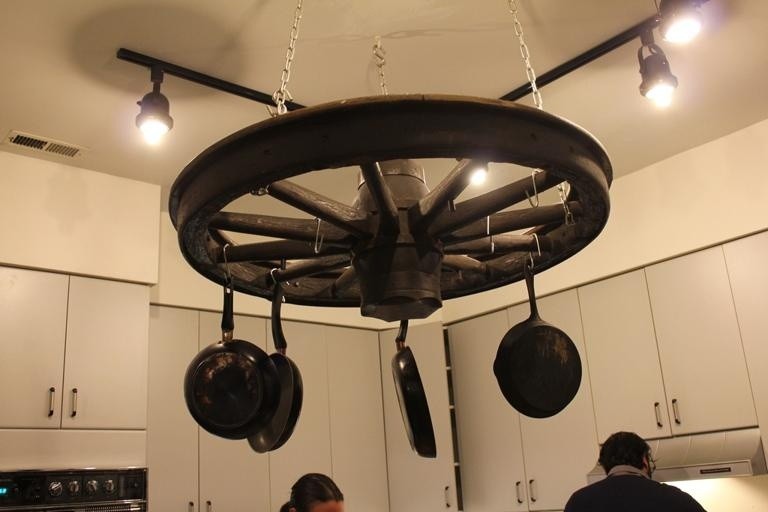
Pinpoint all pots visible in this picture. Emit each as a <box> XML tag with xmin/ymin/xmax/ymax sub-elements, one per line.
<box><xmin>491</xmin><ymin>255</ymin><xmax>585</xmax><ymax>420</ymax></box>
<box><xmin>389</xmin><ymin>317</ymin><xmax>442</xmax><ymax>459</ymax></box>
<box><xmin>181</xmin><ymin>276</ymin><xmax>283</xmax><ymax>440</ymax></box>
<box><xmin>246</xmin><ymin>280</ymin><xmax>305</xmax><ymax>455</ymax></box>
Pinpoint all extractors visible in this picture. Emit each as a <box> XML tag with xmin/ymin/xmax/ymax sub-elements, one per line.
<box><xmin>584</xmin><ymin>425</ymin><xmax>767</xmax><ymax>485</ymax></box>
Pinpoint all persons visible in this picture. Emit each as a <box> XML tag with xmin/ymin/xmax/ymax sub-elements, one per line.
<box><xmin>564</xmin><ymin>431</ymin><xmax>708</xmax><ymax>511</ymax></box>
<box><xmin>279</xmin><ymin>470</ymin><xmax>346</xmax><ymax>512</ymax></box>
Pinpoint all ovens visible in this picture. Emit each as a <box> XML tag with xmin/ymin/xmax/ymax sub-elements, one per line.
<box><xmin>0</xmin><ymin>468</ymin><xmax>148</xmax><ymax>511</ymax></box>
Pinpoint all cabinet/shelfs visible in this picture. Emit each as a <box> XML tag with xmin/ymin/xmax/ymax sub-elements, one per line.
<box><xmin>147</xmin><ymin>303</ymin><xmax>271</xmax><ymax>512</ymax></box>
<box><xmin>266</xmin><ymin>318</ymin><xmax>391</xmax><ymax>512</ymax></box>
<box><xmin>577</xmin><ymin>244</ymin><xmax>758</xmax><ymax>445</ymax></box>
<box><xmin>380</xmin><ymin>321</ymin><xmax>459</xmax><ymax>511</ymax></box>
<box><xmin>0</xmin><ymin>264</ymin><xmax>151</xmax><ymax>471</ymax></box>
<box><xmin>722</xmin><ymin>230</ymin><xmax>768</xmax><ymax>472</ymax></box>
<box><xmin>448</xmin><ymin>289</ymin><xmax>606</xmax><ymax>512</ymax></box>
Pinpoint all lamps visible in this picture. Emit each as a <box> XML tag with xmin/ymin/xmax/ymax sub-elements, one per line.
<box><xmin>116</xmin><ymin>0</ymin><xmax>708</xmax><ymax>185</ymax></box>
